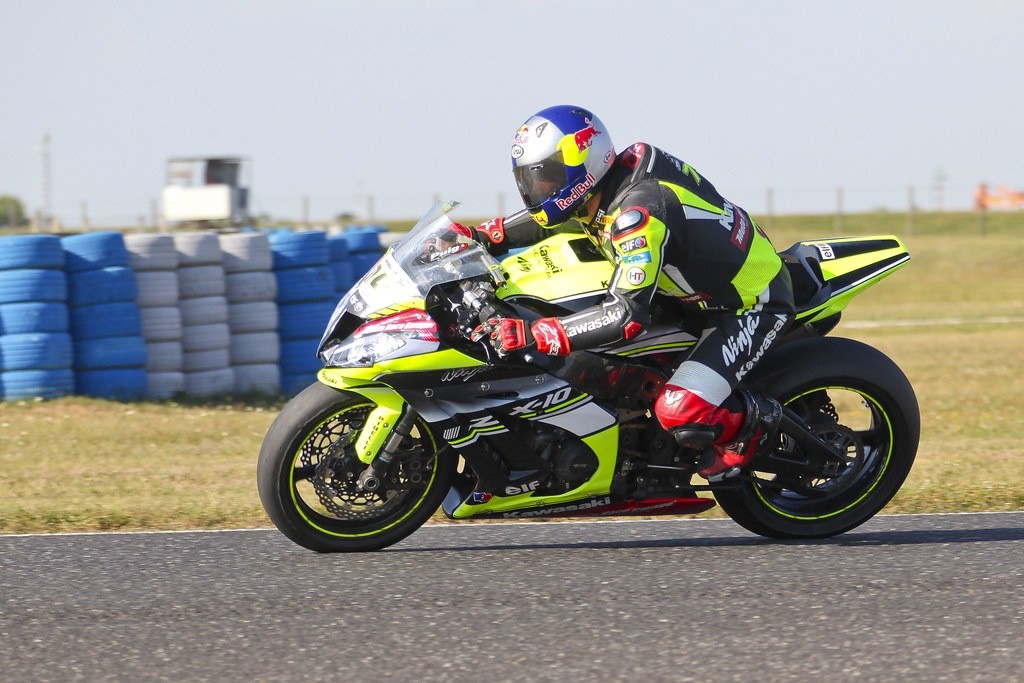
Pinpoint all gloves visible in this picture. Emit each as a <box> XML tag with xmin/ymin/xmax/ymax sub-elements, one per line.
<box><xmin>471</xmin><ymin>317</ymin><xmax>570</xmax><ymax>360</ymax></box>
<box><xmin>422</xmin><ymin>217</ymin><xmax>509</xmax><ymax>264</ymax></box>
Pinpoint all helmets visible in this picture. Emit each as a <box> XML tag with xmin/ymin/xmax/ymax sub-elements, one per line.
<box><xmin>511</xmin><ymin>105</ymin><xmax>615</xmax><ymax>229</ymax></box>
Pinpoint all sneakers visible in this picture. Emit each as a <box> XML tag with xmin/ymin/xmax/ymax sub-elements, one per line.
<box><xmin>697</xmin><ymin>389</ymin><xmax>782</xmax><ymax>482</ymax></box>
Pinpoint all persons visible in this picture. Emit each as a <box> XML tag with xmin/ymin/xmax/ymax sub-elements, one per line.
<box><xmin>425</xmin><ymin>104</ymin><xmax>798</xmax><ymax>483</ymax></box>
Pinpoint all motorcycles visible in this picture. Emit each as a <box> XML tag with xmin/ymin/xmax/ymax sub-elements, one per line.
<box><xmin>254</xmin><ymin>189</ymin><xmax>925</xmax><ymax>557</ymax></box>
